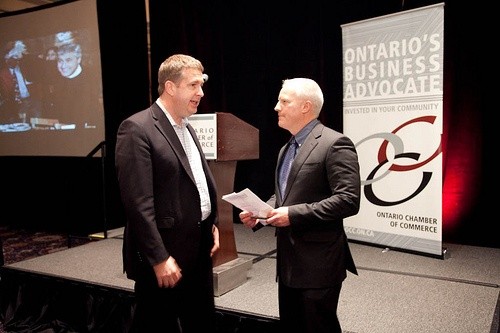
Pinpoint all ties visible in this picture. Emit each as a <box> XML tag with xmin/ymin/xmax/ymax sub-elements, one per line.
<box><xmin>279</xmin><ymin>137</ymin><xmax>297</xmax><ymax>202</ymax></box>
<box><xmin>12</xmin><ymin>72</ymin><xmax>23</xmax><ymax>103</ymax></box>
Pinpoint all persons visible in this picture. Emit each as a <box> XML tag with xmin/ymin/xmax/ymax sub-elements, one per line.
<box><xmin>43</xmin><ymin>47</ymin><xmax>59</xmax><ymax>60</ymax></box>
<box><xmin>116</xmin><ymin>53</ymin><xmax>222</xmax><ymax>333</ymax></box>
<box><xmin>38</xmin><ymin>44</ymin><xmax>99</xmax><ymax>128</ymax></box>
<box><xmin>0</xmin><ymin>41</ymin><xmax>37</xmax><ymax>126</ymax></box>
<box><xmin>238</xmin><ymin>78</ymin><xmax>361</xmax><ymax>333</ymax></box>
